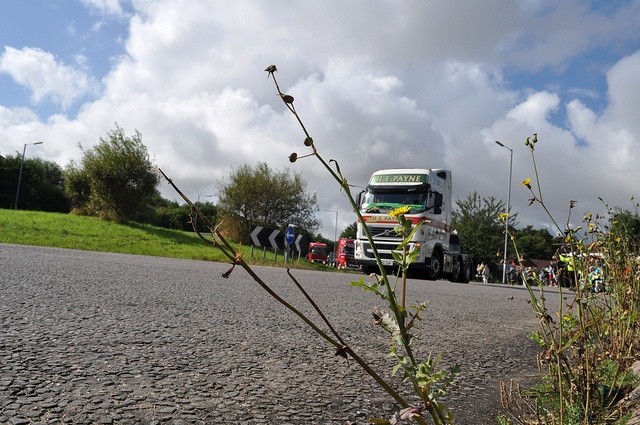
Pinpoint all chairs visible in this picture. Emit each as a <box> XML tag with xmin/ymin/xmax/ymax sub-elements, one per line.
<box><xmin>579</xmin><ymin>271</ymin><xmax>605</xmax><ymax>292</ymax></box>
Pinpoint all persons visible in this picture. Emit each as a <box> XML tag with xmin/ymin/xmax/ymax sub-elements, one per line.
<box><xmin>474</xmin><ymin>252</ymin><xmax>606</xmax><ymax>295</ymax></box>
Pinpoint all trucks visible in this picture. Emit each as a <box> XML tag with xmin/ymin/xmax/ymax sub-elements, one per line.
<box><xmin>305</xmin><ymin>242</ymin><xmax>330</xmax><ymax>267</ymax></box>
<box><xmin>354</xmin><ymin>168</ymin><xmax>476</xmax><ymax>284</ymax></box>
<box><xmin>329</xmin><ymin>238</ymin><xmax>360</xmax><ymax>270</ymax></box>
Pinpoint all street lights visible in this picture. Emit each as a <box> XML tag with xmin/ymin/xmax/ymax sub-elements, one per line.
<box><xmin>194</xmin><ymin>194</ymin><xmax>212</xmax><ymax>232</ymax></box>
<box><xmin>495</xmin><ymin>140</ymin><xmax>513</xmax><ymax>284</ymax></box>
<box><xmin>14</xmin><ymin>141</ymin><xmax>44</xmax><ymax>210</ymax></box>
<box><xmin>324</xmin><ymin>209</ymin><xmax>338</xmax><ymax>262</ymax></box>
